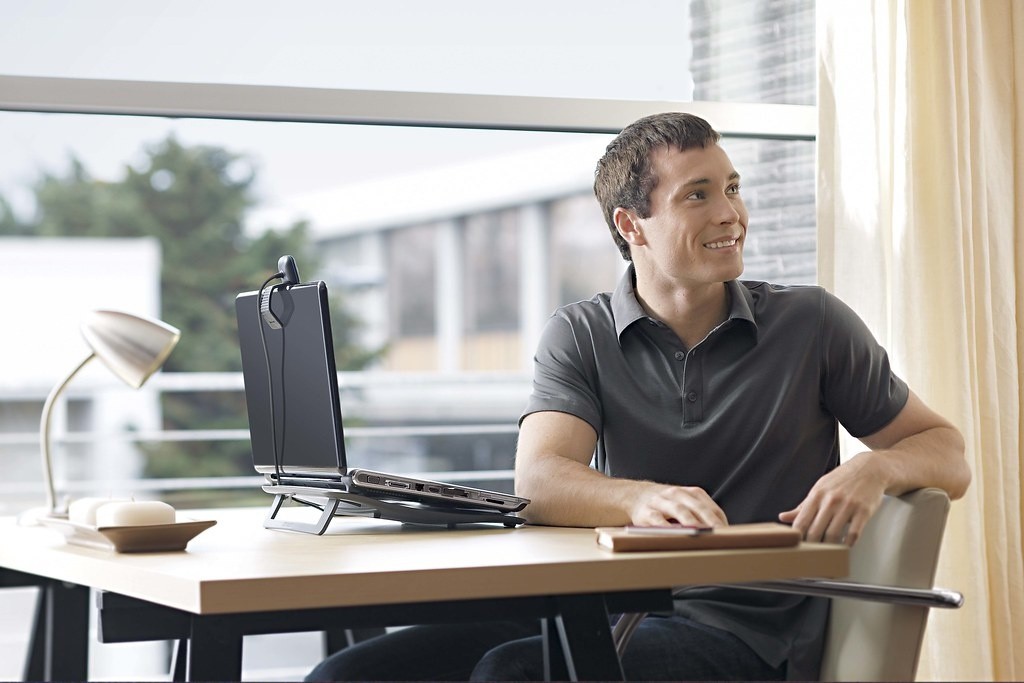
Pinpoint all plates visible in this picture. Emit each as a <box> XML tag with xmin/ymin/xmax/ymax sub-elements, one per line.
<box><xmin>42</xmin><ymin>513</ymin><xmax>218</xmax><ymax>553</ymax></box>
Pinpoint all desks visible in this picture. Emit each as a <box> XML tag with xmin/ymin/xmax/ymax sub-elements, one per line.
<box><xmin>0</xmin><ymin>510</ymin><xmax>848</xmax><ymax>683</ymax></box>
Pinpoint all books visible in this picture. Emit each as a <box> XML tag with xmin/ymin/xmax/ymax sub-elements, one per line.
<box><xmin>594</xmin><ymin>522</ymin><xmax>801</xmax><ymax>550</ymax></box>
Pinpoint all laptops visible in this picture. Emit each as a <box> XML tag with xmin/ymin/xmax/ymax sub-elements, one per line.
<box><xmin>236</xmin><ymin>281</ymin><xmax>532</xmax><ymax>516</ymax></box>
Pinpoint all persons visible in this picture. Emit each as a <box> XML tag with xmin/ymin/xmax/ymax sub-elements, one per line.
<box><xmin>304</xmin><ymin>112</ymin><xmax>973</xmax><ymax>683</ymax></box>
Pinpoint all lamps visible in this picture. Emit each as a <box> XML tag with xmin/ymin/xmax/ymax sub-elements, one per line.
<box><xmin>17</xmin><ymin>310</ymin><xmax>181</xmax><ymax>528</ymax></box>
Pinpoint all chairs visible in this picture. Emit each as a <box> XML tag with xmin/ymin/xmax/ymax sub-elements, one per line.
<box><xmin>822</xmin><ymin>489</ymin><xmax>952</xmax><ymax>683</ymax></box>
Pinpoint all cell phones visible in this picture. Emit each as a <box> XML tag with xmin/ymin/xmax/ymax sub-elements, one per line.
<box><xmin>626</xmin><ymin>524</ymin><xmax>713</xmax><ymax>535</ymax></box>
